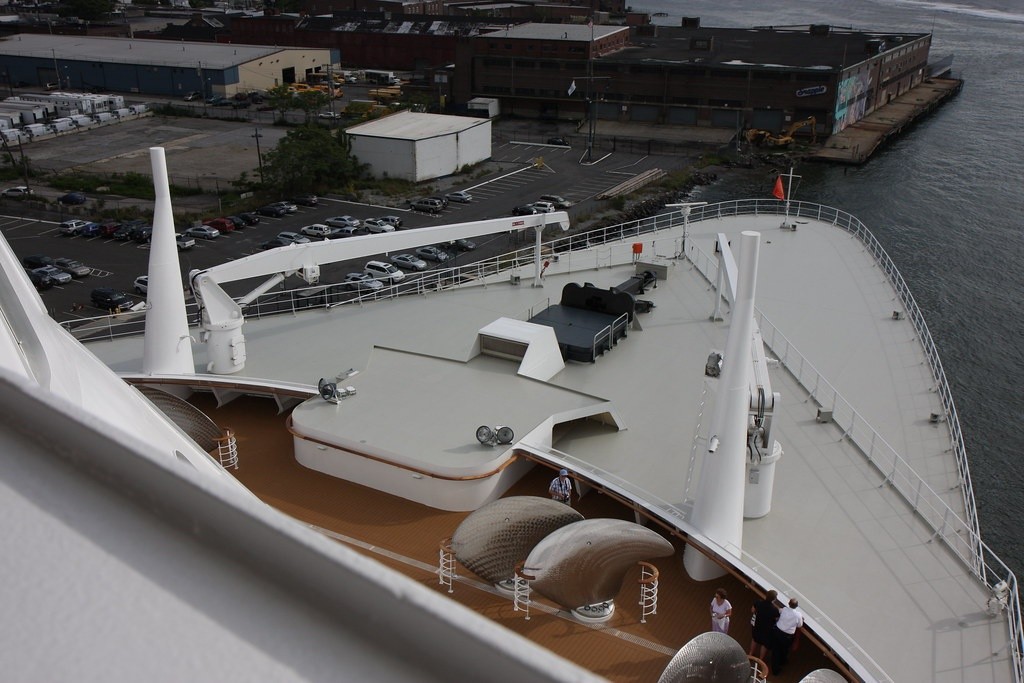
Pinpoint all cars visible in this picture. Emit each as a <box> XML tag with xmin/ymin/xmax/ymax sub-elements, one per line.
<box><xmin>293</xmin><ymin>193</ymin><xmax>317</xmax><ymax>205</ymax></box>
<box><xmin>23</xmin><ymin>256</ymin><xmax>46</xmax><ymax>269</ymax></box>
<box><xmin>89</xmin><ymin>275</ymin><xmax>149</xmax><ymax>311</ymax></box>
<box><xmin>238</xmin><ymin>213</ymin><xmax>259</xmax><ymax>225</ymax></box>
<box><xmin>256</xmin><ymin>205</ymin><xmax>286</xmax><ymax>218</ymax></box>
<box><xmin>184</xmin><ymin>70</ymin><xmax>570</xmax><ymax>146</ymax></box>
<box><xmin>186</xmin><ymin>225</ymin><xmax>220</xmax><ymax>239</ymax></box>
<box><xmin>202</xmin><ymin>218</ymin><xmax>235</xmax><ymax>234</ymax></box>
<box><xmin>99</xmin><ymin>222</ymin><xmax>121</xmax><ymax>237</ymax></box>
<box><xmin>271</xmin><ymin>201</ymin><xmax>298</xmax><ymax>213</ymax></box>
<box><xmin>261</xmin><ymin>192</ymin><xmax>571</xmax><ymax>292</ymax></box>
<box><xmin>34</xmin><ymin>266</ymin><xmax>72</xmax><ymax>285</ymax></box>
<box><xmin>1</xmin><ymin>186</ymin><xmax>100</xmax><ymax>237</ymax></box>
<box><xmin>226</xmin><ymin>216</ymin><xmax>247</xmax><ymax>229</ymax></box>
<box><xmin>54</xmin><ymin>258</ymin><xmax>90</xmax><ymax>279</ymax></box>
<box><xmin>113</xmin><ymin>224</ymin><xmax>152</xmax><ymax>243</ymax></box>
<box><xmin>29</xmin><ymin>273</ymin><xmax>53</xmax><ymax>290</ymax></box>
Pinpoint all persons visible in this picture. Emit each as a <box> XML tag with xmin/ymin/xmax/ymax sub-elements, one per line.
<box><xmin>709</xmin><ymin>588</ymin><xmax>732</xmax><ymax>633</ymax></box>
<box><xmin>771</xmin><ymin>598</ymin><xmax>804</xmax><ymax>676</ymax></box>
<box><xmin>748</xmin><ymin>590</ymin><xmax>780</xmax><ymax>668</ymax></box>
<box><xmin>549</xmin><ymin>469</ymin><xmax>572</xmax><ymax>508</ymax></box>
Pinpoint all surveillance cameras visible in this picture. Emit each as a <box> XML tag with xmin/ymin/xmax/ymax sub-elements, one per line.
<box><xmin>709</xmin><ymin>438</ymin><xmax>718</xmax><ymax>452</ymax></box>
<box><xmin>130</xmin><ymin>302</ymin><xmax>146</xmax><ymax>313</ymax></box>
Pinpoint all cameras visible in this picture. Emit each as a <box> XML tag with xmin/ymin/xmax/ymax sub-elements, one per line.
<box><xmin>712</xmin><ymin>613</ymin><xmax>717</xmax><ymax>616</ymax></box>
<box><xmin>562</xmin><ymin>494</ymin><xmax>569</xmax><ymax>501</ymax></box>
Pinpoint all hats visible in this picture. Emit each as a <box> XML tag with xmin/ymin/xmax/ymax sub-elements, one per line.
<box><xmin>560</xmin><ymin>470</ymin><xmax>568</xmax><ymax>475</ymax></box>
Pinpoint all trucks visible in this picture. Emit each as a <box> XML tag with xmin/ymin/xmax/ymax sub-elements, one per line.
<box><xmin>0</xmin><ymin>80</ymin><xmax>151</xmax><ymax>144</ymax></box>
<box><xmin>175</xmin><ymin>233</ymin><xmax>196</xmax><ymax>251</ymax></box>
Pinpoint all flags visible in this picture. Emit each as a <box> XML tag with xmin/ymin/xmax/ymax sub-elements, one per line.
<box><xmin>568</xmin><ymin>81</ymin><xmax>576</xmax><ymax>96</ymax></box>
<box><xmin>772</xmin><ymin>175</ymin><xmax>784</xmax><ymax>201</ymax></box>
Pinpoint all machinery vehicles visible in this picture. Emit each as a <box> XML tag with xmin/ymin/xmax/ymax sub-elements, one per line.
<box><xmin>745</xmin><ymin>116</ymin><xmax>817</xmax><ymax>150</ymax></box>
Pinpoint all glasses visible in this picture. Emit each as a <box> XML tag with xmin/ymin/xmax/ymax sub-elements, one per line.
<box><xmin>561</xmin><ymin>475</ymin><xmax>567</xmax><ymax>477</ymax></box>
<box><xmin>715</xmin><ymin>597</ymin><xmax>718</xmax><ymax>599</ymax></box>
